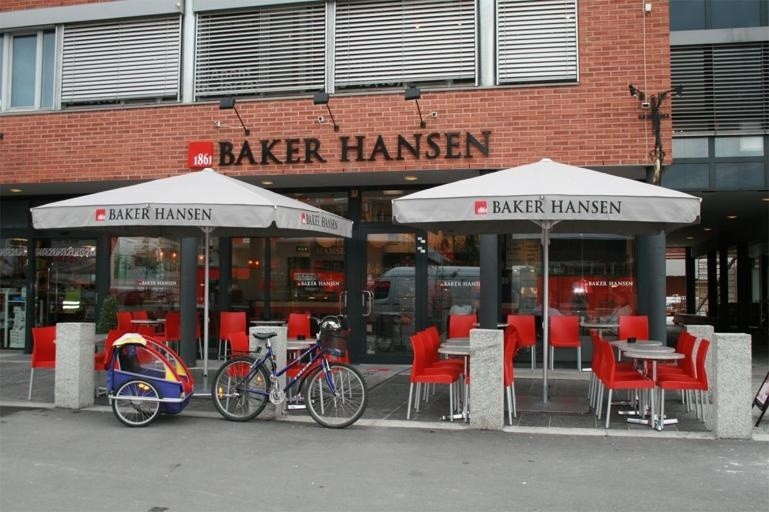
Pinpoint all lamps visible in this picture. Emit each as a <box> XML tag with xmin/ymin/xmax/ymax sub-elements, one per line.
<box><xmin>405</xmin><ymin>85</ymin><xmax>426</xmax><ymax>129</ymax></box>
<box><xmin>314</xmin><ymin>93</ymin><xmax>339</xmax><ymax>131</ymax></box>
<box><xmin>220</xmin><ymin>97</ymin><xmax>250</xmax><ymax>135</ymax></box>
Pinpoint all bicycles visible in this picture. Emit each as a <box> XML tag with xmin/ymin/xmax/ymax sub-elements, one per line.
<box><xmin>213</xmin><ymin>317</ymin><xmax>370</xmax><ymax>429</ymax></box>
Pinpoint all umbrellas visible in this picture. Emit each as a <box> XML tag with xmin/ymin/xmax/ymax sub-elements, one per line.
<box><xmin>392</xmin><ymin>159</ymin><xmax>702</xmax><ymax>414</ymax></box>
<box><xmin>30</xmin><ymin>168</ymin><xmax>353</xmax><ymax>397</ymax></box>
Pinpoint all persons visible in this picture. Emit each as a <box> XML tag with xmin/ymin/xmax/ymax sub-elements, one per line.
<box><xmin>449</xmin><ymin>295</ymin><xmax>473</xmax><ymax>316</ymax></box>
<box><xmin>530</xmin><ymin>293</ymin><xmax>565</xmax><ymax>317</ymax></box>
<box><xmin>606</xmin><ymin>291</ymin><xmax>634</xmax><ymax>336</ymax></box>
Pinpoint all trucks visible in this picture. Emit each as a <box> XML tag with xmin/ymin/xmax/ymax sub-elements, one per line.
<box><xmin>48</xmin><ymin>236</ymin><xmax>182</xmax><ymax>305</ymax></box>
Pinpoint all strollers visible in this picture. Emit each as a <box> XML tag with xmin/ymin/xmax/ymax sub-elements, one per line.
<box><xmin>101</xmin><ymin>332</ymin><xmax>198</xmax><ymax>422</ymax></box>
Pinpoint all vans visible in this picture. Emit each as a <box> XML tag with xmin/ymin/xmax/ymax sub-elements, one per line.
<box><xmin>367</xmin><ymin>265</ymin><xmax>539</xmax><ymax>350</ymax></box>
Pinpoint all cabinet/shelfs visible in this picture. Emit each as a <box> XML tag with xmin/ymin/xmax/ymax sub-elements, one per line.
<box><xmin>0</xmin><ymin>287</ymin><xmax>26</xmax><ymax>349</ymax></box>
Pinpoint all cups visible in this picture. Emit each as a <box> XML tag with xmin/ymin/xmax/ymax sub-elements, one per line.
<box><xmin>297</xmin><ymin>335</ymin><xmax>305</xmax><ymax>340</ymax></box>
<box><xmin>151</xmin><ymin>317</ymin><xmax>157</xmax><ymax>320</ymax></box>
<box><xmin>626</xmin><ymin>336</ymin><xmax>635</xmax><ymax>343</ymax></box>
